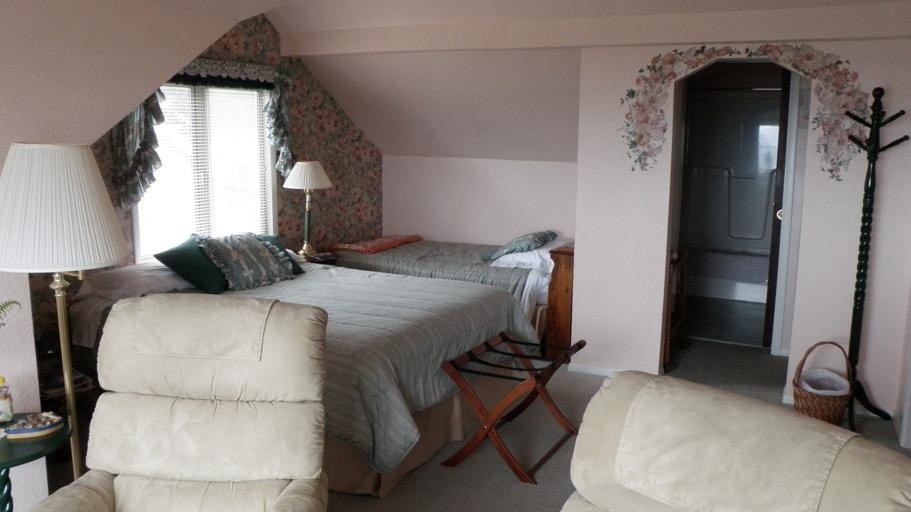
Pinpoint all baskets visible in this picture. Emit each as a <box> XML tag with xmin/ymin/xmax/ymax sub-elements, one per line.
<box><xmin>791</xmin><ymin>341</ymin><xmax>854</xmax><ymax>425</ymax></box>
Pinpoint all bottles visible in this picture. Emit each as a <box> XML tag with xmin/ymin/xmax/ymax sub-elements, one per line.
<box><xmin>0</xmin><ymin>377</ymin><xmax>15</xmax><ymax>424</ymax></box>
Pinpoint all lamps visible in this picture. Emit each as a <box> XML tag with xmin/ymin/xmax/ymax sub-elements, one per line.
<box><xmin>1</xmin><ymin>140</ymin><xmax>131</xmax><ymax>480</ymax></box>
<box><xmin>283</xmin><ymin>160</ymin><xmax>334</xmax><ymax>255</ymax></box>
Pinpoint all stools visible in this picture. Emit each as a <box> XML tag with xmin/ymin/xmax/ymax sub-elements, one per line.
<box><xmin>437</xmin><ymin>328</ymin><xmax>590</xmax><ymax>487</ymax></box>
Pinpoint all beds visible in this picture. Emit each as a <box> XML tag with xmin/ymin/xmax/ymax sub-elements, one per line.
<box><xmin>70</xmin><ymin>232</ymin><xmax>540</xmax><ymax>494</ymax></box>
<box><xmin>311</xmin><ymin>233</ymin><xmax>573</xmax><ymax>350</ymax></box>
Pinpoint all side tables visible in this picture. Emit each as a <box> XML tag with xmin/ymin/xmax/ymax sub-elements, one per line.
<box><xmin>2</xmin><ymin>412</ymin><xmax>73</xmax><ymax>512</ymax></box>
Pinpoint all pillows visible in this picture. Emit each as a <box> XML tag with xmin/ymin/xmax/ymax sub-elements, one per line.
<box><xmin>488</xmin><ymin>229</ymin><xmax>557</xmax><ymax>263</ymax></box>
<box><xmin>152</xmin><ymin>232</ymin><xmax>306</xmax><ymax>293</ymax></box>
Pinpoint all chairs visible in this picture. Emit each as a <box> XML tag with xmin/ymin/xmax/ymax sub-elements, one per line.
<box><xmin>23</xmin><ymin>290</ymin><xmax>336</xmax><ymax>512</ymax></box>
